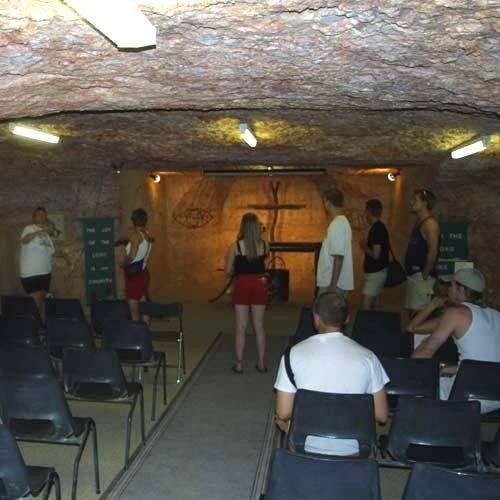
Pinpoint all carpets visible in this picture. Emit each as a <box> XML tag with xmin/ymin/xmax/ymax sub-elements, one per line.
<box><xmin>93</xmin><ymin>328</ymin><xmax>298</xmax><ymax>500</ymax></box>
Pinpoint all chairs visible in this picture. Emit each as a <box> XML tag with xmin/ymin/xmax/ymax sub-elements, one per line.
<box><xmin>263</xmin><ymin>309</ymin><xmax>500</xmax><ymax>499</ymax></box>
<box><xmin>0</xmin><ymin>294</ymin><xmax>188</xmax><ymax>499</ymax></box>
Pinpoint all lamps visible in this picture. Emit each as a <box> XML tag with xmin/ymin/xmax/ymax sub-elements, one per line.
<box><xmin>448</xmin><ymin>132</ymin><xmax>491</xmax><ymax>160</ymax></box>
<box><xmin>55</xmin><ymin>0</ymin><xmax>161</xmax><ymax>53</ymax></box>
<box><xmin>8</xmin><ymin>121</ymin><xmax>60</xmax><ymax>149</ymax></box>
<box><xmin>238</xmin><ymin>119</ymin><xmax>258</xmax><ymax>149</ymax></box>
<box><xmin>386</xmin><ymin>169</ymin><xmax>401</xmax><ymax>183</ymax></box>
<box><xmin>147</xmin><ymin>170</ymin><xmax>162</xmax><ymax>185</ymax></box>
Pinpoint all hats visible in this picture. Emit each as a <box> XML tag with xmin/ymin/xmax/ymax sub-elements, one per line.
<box><xmin>441</xmin><ymin>268</ymin><xmax>486</xmax><ymax>293</ymax></box>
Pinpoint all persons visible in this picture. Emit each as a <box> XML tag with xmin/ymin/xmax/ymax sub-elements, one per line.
<box><xmin>403</xmin><ymin>188</ymin><xmax>441</xmax><ymax>323</ymax></box>
<box><xmin>406</xmin><ymin>268</ymin><xmax>500</xmax><ymax>413</ymax></box>
<box><xmin>18</xmin><ymin>207</ymin><xmax>56</xmax><ymax>323</ymax></box>
<box><xmin>272</xmin><ymin>292</ymin><xmax>389</xmax><ymax>438</ymax></box>
<box><xmin>226</xmin><ymin>213</ymin><xmax>272</xmax><ymax>373</ymax></box>
<box><xmin>119</xmin><ymin>207</ymin><xmax>154</xmax><ymax>329</ymax></box>
<box><xmin>316</xmin><ymin>189</ymin><xmax>355</xmax><ymax>306</ymax></box>
<box><xmin>358</xmin><ymin>198</ymin><xmax>390</xmax><ymax>312</ymax></box>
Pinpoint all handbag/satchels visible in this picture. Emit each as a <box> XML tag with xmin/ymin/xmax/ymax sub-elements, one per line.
<box><xmin>124</xmin><ymin>258</ymin><xmax>143</xmax><ymax>276</ymax></box>
<box><xmin>384</xmin><ymin>261</ymin><xmax>407</xmax><ymax>287</ymax></box>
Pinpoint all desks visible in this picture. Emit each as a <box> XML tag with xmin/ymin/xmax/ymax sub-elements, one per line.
<box><xmin>266</xmin><ymin>241</ymin><xmax>324</xmax><ymax>306</ymax></box>
